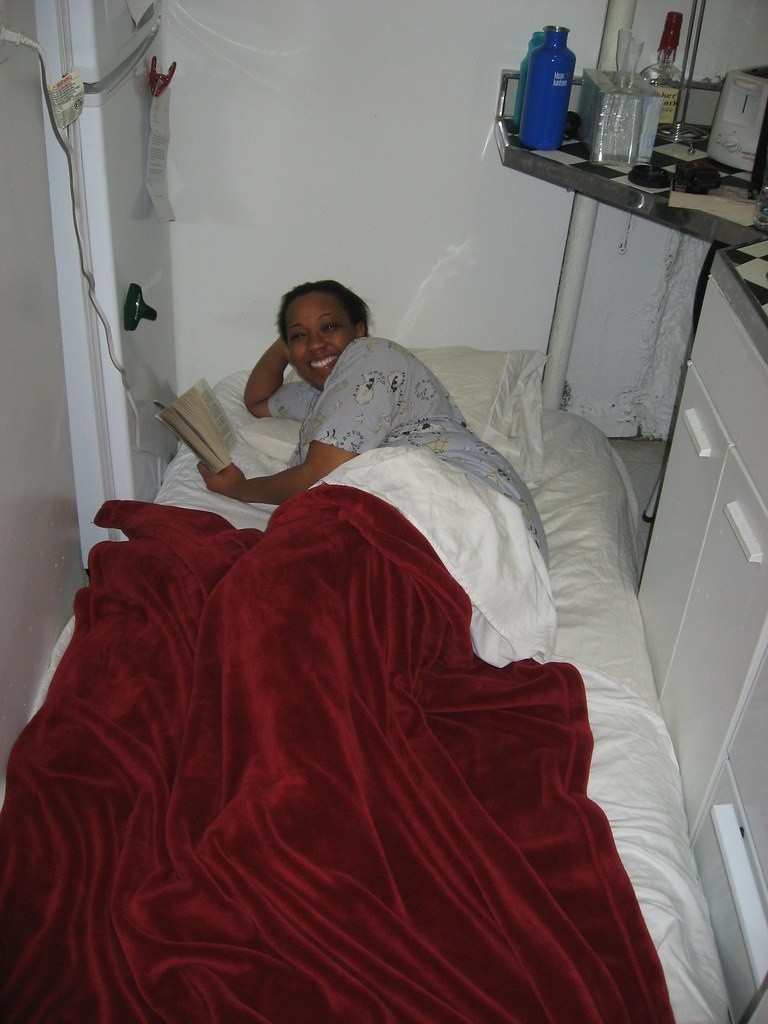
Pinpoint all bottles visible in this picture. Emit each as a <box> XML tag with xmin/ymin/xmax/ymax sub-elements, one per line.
<box><xmin>639</xmin><ymin>12</ymin><xmax>684</xmax><ymax>136</ymax></box>
<box><xmin>518</xmin><ymin>25</ymin><xmax>576</xmax><ymax>150</ymax></box>
<box><xmin>513</xmin><ymin>32</ymin><xmax>546</xmax><ymax>133</ymax></box>
<box><xmin>753</xmin><ymin>162</ymin><xmax>768</xmax><ymax>233</ymax></box>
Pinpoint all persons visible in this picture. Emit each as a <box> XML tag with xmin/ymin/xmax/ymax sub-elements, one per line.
<box><xmin>196</xmin><ymin>280</ymin><xmax>558</xmax><ymax>667</ymax></box>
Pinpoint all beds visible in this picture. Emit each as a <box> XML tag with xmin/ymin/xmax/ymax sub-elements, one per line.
<box><xmin>0</xmin><ymin>409</ymin><xmax>728</xmax><ymax>1024</ymax></box>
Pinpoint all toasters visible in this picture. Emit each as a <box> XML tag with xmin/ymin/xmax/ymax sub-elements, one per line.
<box><xmin>706</xmin><ymin>64</ymin><xmax>768</xmax><ymax>171</ymax></box>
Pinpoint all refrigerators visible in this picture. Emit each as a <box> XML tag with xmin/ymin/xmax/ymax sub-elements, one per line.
<box><xmin>35</xmin><ymin>0</ymin><xmax>183</xmax><ymax>576</ymax></box>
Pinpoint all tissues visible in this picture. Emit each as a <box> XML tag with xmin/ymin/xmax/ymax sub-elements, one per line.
<box><xmin>576</xmin><ymin>30</ymin><xmax>665</xmax><ymax>166</ymax></box>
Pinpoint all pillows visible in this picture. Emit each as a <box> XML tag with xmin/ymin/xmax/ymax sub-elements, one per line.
<box><xmin>235</xmin><ymin>346</ymin><xmax>547</xmax><ymax>490</ymax></box>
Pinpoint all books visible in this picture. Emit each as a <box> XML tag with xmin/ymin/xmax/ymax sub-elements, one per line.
<box><xmin>155</xmin><ymin>377</ymin><xmax>238</xmax><ymax>475</ymax></box>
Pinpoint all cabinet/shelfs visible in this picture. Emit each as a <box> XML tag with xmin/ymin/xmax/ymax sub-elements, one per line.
<box><xmin>626</xmin><ymin>275</ymin><xmax>768</xmax><ymax>1023</ymax></box>
